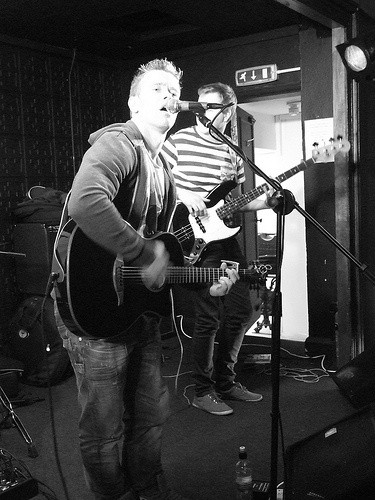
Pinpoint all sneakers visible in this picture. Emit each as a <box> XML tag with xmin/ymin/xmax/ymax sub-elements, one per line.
<box><xmin>192</xmin><ymin>392</ymin><xmax>233</xmax><ymax>415</ymax></box>
<box><xmin>221</xmin><ymin>382</ymin><xmax>263</xmax><ymax>401</ymax></box>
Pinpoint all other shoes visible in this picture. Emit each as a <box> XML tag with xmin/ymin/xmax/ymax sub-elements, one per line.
<box><xmin>118</xmin><ymin>472</ymin><xmax>189</xmax><ymax>500</ymax></box>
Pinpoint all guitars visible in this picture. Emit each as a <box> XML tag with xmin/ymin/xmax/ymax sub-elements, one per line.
<box><xmin>53</xmin><ymin>218</ymin><xmax>267</xmax><ymax>340</ymax></box>
<box><xmin>168</xmin><ymin>134</ymin><xmax>351</xmax><ymax>267</ymax></box>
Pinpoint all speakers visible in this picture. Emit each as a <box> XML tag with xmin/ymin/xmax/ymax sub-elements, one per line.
<box><xmin>280</xmin><ymin>344</ymin><xmax>375</xmax><ymax>500</ymax></box>
<box><xmin>14</xmin><ymin>223</ymin><xmax>63</xmax><ymax>295</ymax></box>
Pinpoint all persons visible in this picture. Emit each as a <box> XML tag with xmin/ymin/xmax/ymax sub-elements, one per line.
<box><xmin>48</xmin><ymin>59</ymin><xmax>242</xmax><ymax>499</ymax></box>
<box><xmin>160</xmin><ymin>82</ymin><xmax>294</xmax><ymax>416</ymax></box>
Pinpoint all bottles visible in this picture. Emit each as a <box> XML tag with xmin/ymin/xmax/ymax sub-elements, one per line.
<box><xmin>236</xmin><ymin>445</ymin><xmax>253</xmax><ymax>500</ymax></box>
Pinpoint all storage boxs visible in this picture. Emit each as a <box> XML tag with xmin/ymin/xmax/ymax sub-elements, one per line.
<box><xmin>12</xmin><ymin>223</ymin><xmax>60</xmax><ymax>296</ymax></box>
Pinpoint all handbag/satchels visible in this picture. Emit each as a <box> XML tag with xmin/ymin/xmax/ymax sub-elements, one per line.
<box><xmin>0</xmin><ymin>357</ymin><xmax>24</xmax><ymax>401</ymax></box>
<box><xmin>15</xmin><ymin>186</ymin><xmax>68</xmax><ymax>225</ymax></box>
<box><xmin>4</xmin><ymin>296</ymin><xmax>70</xmax><ymax>386</ymax></box>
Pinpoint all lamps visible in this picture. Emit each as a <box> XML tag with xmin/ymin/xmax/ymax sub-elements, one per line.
<box><xmin>335</xmin><ymin>28</ymin><xmax>375</xmax><ymax>84</ymax></box>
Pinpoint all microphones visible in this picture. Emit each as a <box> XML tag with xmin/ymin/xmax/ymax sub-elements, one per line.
<box><xmin>166</xmin><ymin>99</ymin><xmax>229</xmax><ymax>114</ymax></box>
